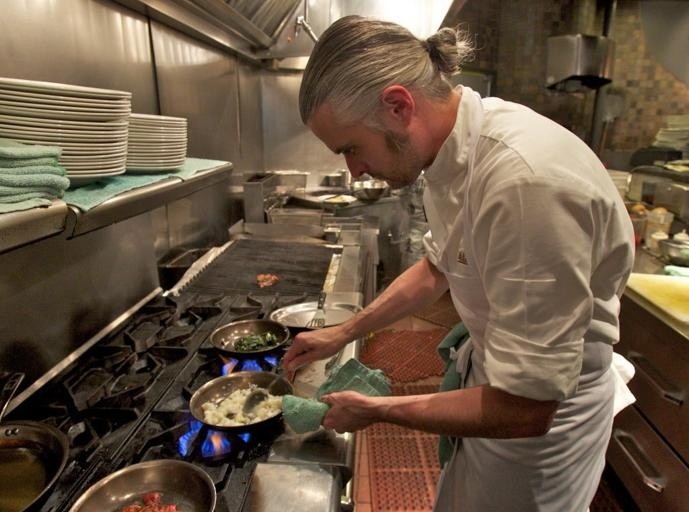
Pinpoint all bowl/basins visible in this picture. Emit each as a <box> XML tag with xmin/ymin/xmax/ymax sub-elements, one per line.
<box><xmin>350</xmin><ymin>178</ymin><xmax>390</xmax><ymax>202</ymax></box>
<box><xmin>70</xmin><ymin>458</ymin><xmax>218</xmax><ymax>511</ymax></box>
<box><xmin>660</xmin><ymin>238</ymin><xmax>689</xmax><ymax>266</ymax></box>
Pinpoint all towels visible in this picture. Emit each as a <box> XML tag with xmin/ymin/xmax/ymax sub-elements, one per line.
<box><xmin>62</xmin><ymin>176</ymin><xmax>170</xmax><ymax>212</ymax></box>
<box><xmin>172</xmin><ymin>159</ymin><xmax>228</xmax><ymax>182</ymax></box>
<box><xmin>285</xmin><ymin>358</ymin><xmax>393</xmax><ymax>434</ymax></box>
<box><xmin>0</xmin><ymin>138</ymin><xmax>72</xmax><ymax>213</ymax></box>
<box><xmin>437</xmin><ymin>322</ymin><xmax>470</xmax><ymax>468</ymax></box>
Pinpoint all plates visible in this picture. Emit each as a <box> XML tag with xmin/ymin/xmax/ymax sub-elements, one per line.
<box><xmin>127</xmin><ymin>112</ymin><xmax>187</xmax><ymax>174</ymax></box>
<box><xmin>0</xmin><ymin>76</ymin><xmax>132</xmax><ymax>184</ymax></box>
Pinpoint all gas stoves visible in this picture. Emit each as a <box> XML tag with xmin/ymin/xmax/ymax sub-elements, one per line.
<box><xmin>5</xmin><ymin>285</ymin><xmax>326</xmax><ymax>512</ymax></box>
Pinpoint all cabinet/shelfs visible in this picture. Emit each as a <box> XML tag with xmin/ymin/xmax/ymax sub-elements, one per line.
<box><xmin>600</xmin><ymin>294</ymin><xmax>688</xmax><ymax>506</ymax></box>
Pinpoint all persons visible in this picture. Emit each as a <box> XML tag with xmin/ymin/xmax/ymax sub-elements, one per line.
<box><xmin>283</xmin><ymin>15</ymin><xmax>637</xmax><ymax>512</ymax></box>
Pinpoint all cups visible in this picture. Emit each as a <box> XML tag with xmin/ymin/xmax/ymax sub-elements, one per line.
<box><xmin>646</xmin><ymin>210</ymin><xmax>689</xmax><ymax>253</ymax></box>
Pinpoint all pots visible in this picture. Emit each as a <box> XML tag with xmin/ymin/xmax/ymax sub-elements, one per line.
<box><xmin>1</xmin><ymin>370</ymin><xmax>71</xmax><ymax>512</ymax></box>
<box><xmin>188</xmin><ymin>371</ymin><xmax>295</xmax><ymax>428</ymax></box>
<box><xmin>209</xmin><ymin>319</ymin><xmax>291</xmax><ymax>354</ymax></box>
